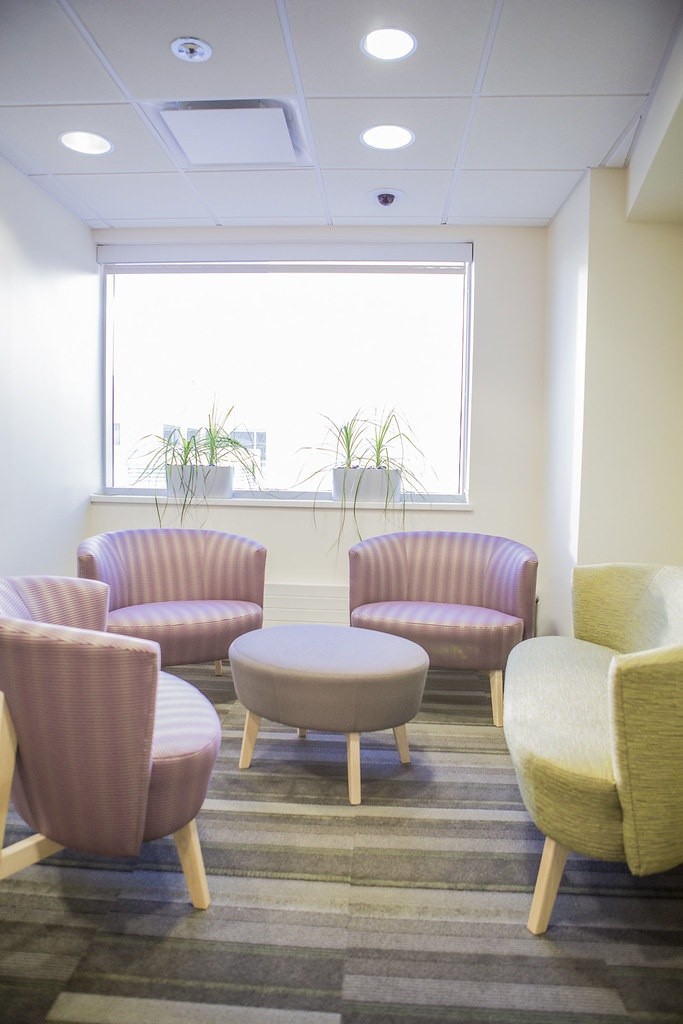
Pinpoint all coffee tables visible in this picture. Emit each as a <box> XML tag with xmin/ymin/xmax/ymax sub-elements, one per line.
<box><xmin>228</xmin><ymin>624</ymin><xmax>431</xmax><ymax>808</ymax></box>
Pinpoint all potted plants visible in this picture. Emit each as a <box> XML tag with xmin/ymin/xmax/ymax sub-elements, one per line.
<box><xmin>135</xmin><ymin>403</ymin><xmax>261</xmax><ymax>528</ymax></box>
<box><xmin>298</xmin><ymin>408</ymin><xmax>426</xmax><ymax>552</ymax></box>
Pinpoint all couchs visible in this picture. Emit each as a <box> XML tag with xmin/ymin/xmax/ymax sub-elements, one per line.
<box><xmin>501</xmin><ymin>564</ymin><xmax>683</xmax><ymax>934</ymax></box>
<box><xmin>0</xmin><ymin>576</ymin><xmax>223</xmax><ymax>910</ymax></box>
<box><xmin>347</xmin><ymin>530</ymin><xmax>541</xmax><ymax>724</ymax></box>
<box><xmin>76</xmin><ymin>528</ymin><xmax>267</xmax><ymax>676</ymax></box>
